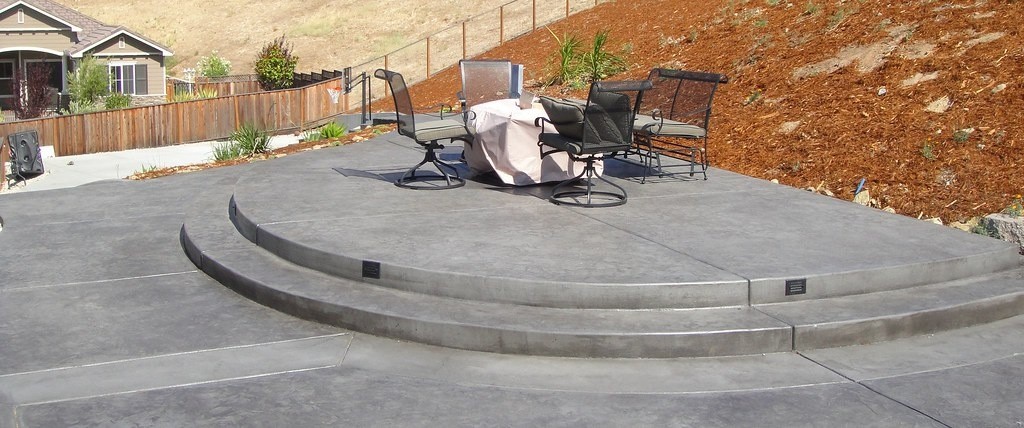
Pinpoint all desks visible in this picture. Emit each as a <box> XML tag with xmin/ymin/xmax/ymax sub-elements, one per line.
<box><xmin>464</xmin><ymin>98</ymin><xmax>605</xmax><ymax>186</ymax></box>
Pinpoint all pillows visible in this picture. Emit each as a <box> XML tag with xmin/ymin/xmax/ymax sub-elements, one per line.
<box><xmin>539</xmin><ymin>84</ymin><xmax>630</xmax><ymax>144</ymax></box>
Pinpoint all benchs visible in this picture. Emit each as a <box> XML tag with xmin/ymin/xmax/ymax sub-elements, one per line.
<box><xmin>624</xmin><ymin>67</ymin><xmax>728</xmax><ymax>185</ymax></box>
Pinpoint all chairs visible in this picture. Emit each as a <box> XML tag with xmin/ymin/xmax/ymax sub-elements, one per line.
<box><xmin>374</xmin><ymin>69</ymin><xmax>469</xmax><ymax>190</ymax></box>
<box><xmin>457</xmin><ymin>60</ymin><xmax>521</xmax><ymax>113</ymax></box>
<box><xmin>535</xmin><ymin>80</ymin><xmax>653</xmax><ymax>207</ymax></box>
